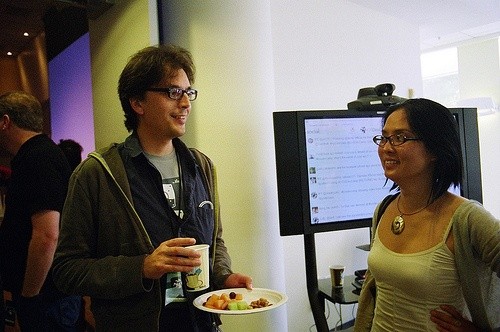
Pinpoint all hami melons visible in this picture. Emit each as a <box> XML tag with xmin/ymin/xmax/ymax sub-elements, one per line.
<box><xmin>205</xmin><ymin>293</ymin><xmax>242</xmax><ymax>309</ymax></box>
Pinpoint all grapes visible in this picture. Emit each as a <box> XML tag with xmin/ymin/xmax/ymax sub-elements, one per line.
<box><xmin>229</xmin><ymin>292</ymin><xmax>236</xmax><ymax>299</ymax></box>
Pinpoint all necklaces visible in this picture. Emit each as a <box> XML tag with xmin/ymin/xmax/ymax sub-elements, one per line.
<box><xmin>391</xmin><ymin>194</ymin><xmax>433</xmax><ymax>235</ymax></box>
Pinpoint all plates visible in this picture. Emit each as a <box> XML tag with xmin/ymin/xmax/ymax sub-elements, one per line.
<box><xmin>193</xmin><ymin>288</ymin><xmax>288</xmax><ymax>315</ymax></box>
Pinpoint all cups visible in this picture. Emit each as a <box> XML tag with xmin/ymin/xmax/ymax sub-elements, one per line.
<box><xmin>181</xmin><ymin>244</ymin><xmax>210</xmax><ymax>293</ymax></box>
<box><xmin>330</xmin><ymin>265</ymin><xmax>345</xmax><ymax>288</ymax></box>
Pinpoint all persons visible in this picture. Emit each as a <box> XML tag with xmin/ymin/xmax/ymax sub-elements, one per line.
<box><xmin>354</xmin><ymin>98</ymin><xmax>500</xmax><ymax>332</ymax></box>
<box><xmin>50</xmin><ymin>45</ymin><xmax>252</xmax><ymax>332</ymax></box>
<box><xmin>0</xmin><ymin>91</ymin><xmax>83</xmax><ymax>332</ymax></box>
<box><xmin>56</xmin><ymin>139</ymin><xmax>82</xmax><ymax>169</ymax></box>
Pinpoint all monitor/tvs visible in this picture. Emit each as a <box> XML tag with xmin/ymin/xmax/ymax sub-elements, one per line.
<box><xmin>271</xmin><ymin>107</ymin><xmax>483</xmax><ymax>236</ymax></box>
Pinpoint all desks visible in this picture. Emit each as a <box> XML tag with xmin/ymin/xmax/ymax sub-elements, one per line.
<box><xmin>317</xmin><ymin>275</ymin><xmax>360</xmax><ymax>311</ymax></box>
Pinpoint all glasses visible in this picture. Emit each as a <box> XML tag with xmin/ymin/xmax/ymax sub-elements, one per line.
<box><xmin>373</xmin><ymin>135</ymin><xmax>422</xmax><ymax>147</ymax></box>
<box><xmin>144</xmin><ymin>87</ymin><xmax>197</xmax><ymax>101</ymax></box>
